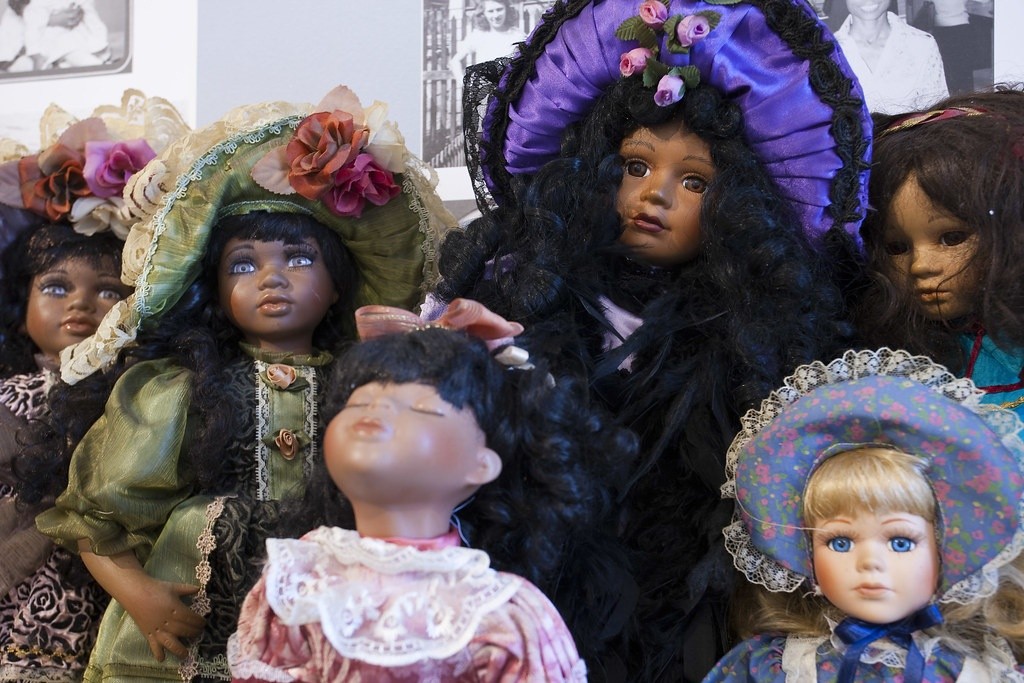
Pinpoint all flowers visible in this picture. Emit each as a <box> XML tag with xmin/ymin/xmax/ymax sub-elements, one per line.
<box><xmin>250</xmin><ymin>84</ymin><xmax>407</xmax><ymax>219</ymax></box>
<box><xmin>614</xmin><ymin>0</ymin><xmax>722</xmax><ymax>107</ymax></box>
<box><xmin>260</xmin><ymin>364</ymin><xmax>311</xmax><ymax>393</ymax></box>
<box><xmin>262</xmin><ymin>429</ymin><xmax>328</xmax><ymax>460</ymax></box>
<box><xmin>0</xmin><ymin>116</ymin><xmax>156</xmax><ymax>240</ymax></box>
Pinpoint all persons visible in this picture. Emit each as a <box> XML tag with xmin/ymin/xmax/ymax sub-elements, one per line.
<box><xmin>227</xmin><ymin>329</ymin><xmax>587</xmax><ymax>682</ymax></box>
<box><xmin>0</xmin><ymin>2</ymin><xmax>1024</xmax><ymax>683</ymax></box>
